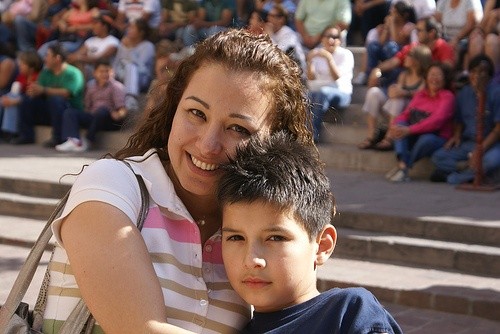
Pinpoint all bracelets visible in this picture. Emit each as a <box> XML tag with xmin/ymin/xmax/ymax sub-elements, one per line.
<box><xmin>376</xmin><ymin>67</ymin><xmax>382</xmax><ymax>78</ymax></box>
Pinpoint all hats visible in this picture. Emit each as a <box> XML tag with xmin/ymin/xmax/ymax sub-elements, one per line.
<box><xmin>92</xmin><ymin>11</ymin><xmax>114</xmax><ymax>28</ymax></box>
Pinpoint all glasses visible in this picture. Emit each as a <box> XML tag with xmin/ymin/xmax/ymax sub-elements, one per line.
<box><xmin>324</xmin><ymin>33</ymin><xmax>339</xmax><ymax>39</ymax></box>
<box><xmin>268</xmin><ymin>13</ymin><xmax>276</xmax><ymax>18</ymax></box>
<box><xmin>406</xmin><ymin>51</ymin><xmax>415</xmax><ymax>59</ymax></box>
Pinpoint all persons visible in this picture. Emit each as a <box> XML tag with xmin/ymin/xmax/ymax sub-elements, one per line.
<box><xmin>113</xmin><ymin>18</ymin><xmax>155</xmax><ymax>110</ymax></box>
<box><xmin>0</xmin><ymin>0</ymin><xmax>355</xmax><ymax>147</ymax></box>
<box><xmin>39</xmin><ymin>30</ymin><xmax>312</xmax><ymax>334</ymax></box>
<box><xmin>219</xmin><ymin>131</ymin><xmax>402</xmax><ymax>334</ymax></box>
<box><xmin>352</xmin><ymin>0</ymin><xmax>500</xmax><ymax>185</ymax></box>
<box><xmin>68</xmin><ymin>14</ymin><xmax>119</xmax><ymax>78</ymax></box>
<box><xmin>54</xmin><ymin>59</ymin><xmax>128</xmax><ymax>151</ymax></box>
<box><xmin>264</xmin><ymin>7</ymin><xmax>307</xmax><ymax>77</ymax></box>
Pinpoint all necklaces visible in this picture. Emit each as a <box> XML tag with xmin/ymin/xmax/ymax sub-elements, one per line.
<box><xmin>196</xmin><ymin>219</ymin><xmax>206</xmax><ymax>226</ymax></box>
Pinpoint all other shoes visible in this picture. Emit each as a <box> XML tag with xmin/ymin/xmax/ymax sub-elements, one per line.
<box><xmin>11</xmin><ymin>135</ymin><xmax>34</xmax><ymax>144</ymax></box>
<box><xmin>360</xmin><ymin>137</ymin><xmax>376</xmax><ymax>151</ymax></box>
<box><xmin>392</xmin><ymin>170</ymin><xmax>408</xmax><ymax>182</ymax></box>
<box><xmin>354</xmin><ymin>72</ymin><xmax>365</xmax><ymax>84</ymax></box>
<box><xmin>56</xmin><ymin>139</ymin><xmax>81</xmax><ymax>151</ymax></box>
<box><xmin>456</xmin><ymin>75</ymin><xmax>469</xmax><ymax>88</ymax></box>
<box><xmin>430</xmin><ymin>169</ymin><xmax>451</xmax><ymax>183</ymax></box>
<box><xmin>42</xmin><ymin>135</ymin><xmax>61</xmax><ymax>148</ymax></box>
<box><xmin>385</xmin><ymin>167</ymin><xmax>398</xmax><ymax>179</ymax></box>
<box><xmin>374</xmin><ymin>138</ymin><xmax>392</xmax><ymax>150</ymax></box>
<box><xmin>72</xmin><ymin>140</ymin><xmax>90</xmax><ymax>152</ymax></box>
